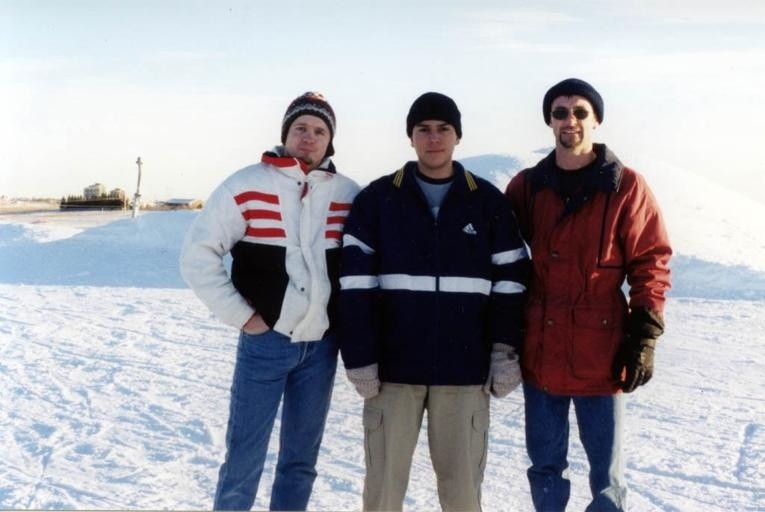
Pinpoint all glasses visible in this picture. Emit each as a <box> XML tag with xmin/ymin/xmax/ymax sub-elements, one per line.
<box><xmin>548</xmin><ymin>103</ymin><xmax>595</xmax><ymax>121</ymax></box>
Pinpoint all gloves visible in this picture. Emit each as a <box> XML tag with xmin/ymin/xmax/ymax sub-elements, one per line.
<box><xmin>483</xmin><ymin>340</ymin><xmax>525</xmax><ymax>398</ymax></box>
<box><xmin>613</xmin><ymin>306</ymin><xmax>666</xmax><ymax>394</ymax></box>
<box><xmin>344</xmin><ymin>359</ymin><xmax>383</xmax><ymax>400</ymax></box>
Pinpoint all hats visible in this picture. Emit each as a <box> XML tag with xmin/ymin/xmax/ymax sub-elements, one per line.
<box><xmin>541</xmin><ymin>76</ymin><xmax>606</xmax><ymax>126</ymax></box>
<box><xmin>279</xmin><ymin>88</ymin><xmax>338</xmax><ymax>154</ymax></box>
<box><xmin>405</xmin><ymin>91</ymin><xmax>464</xmax><ymax>139</ymax></box>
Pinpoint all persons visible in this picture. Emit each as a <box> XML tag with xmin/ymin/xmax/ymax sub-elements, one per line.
<box><xmin>179</xmin><ymin>91</ymin><xmax>361</xmax><ymax>512</ymax></box>
<box><xmin>505</xmin><ymin>78</ymin><xmax>673</xmax><ymax>512</ymax></box>
<box><xmin>330</xmin><ymin>92</ymin><xmax>531</xmax><ymax>512</ymax></box>
